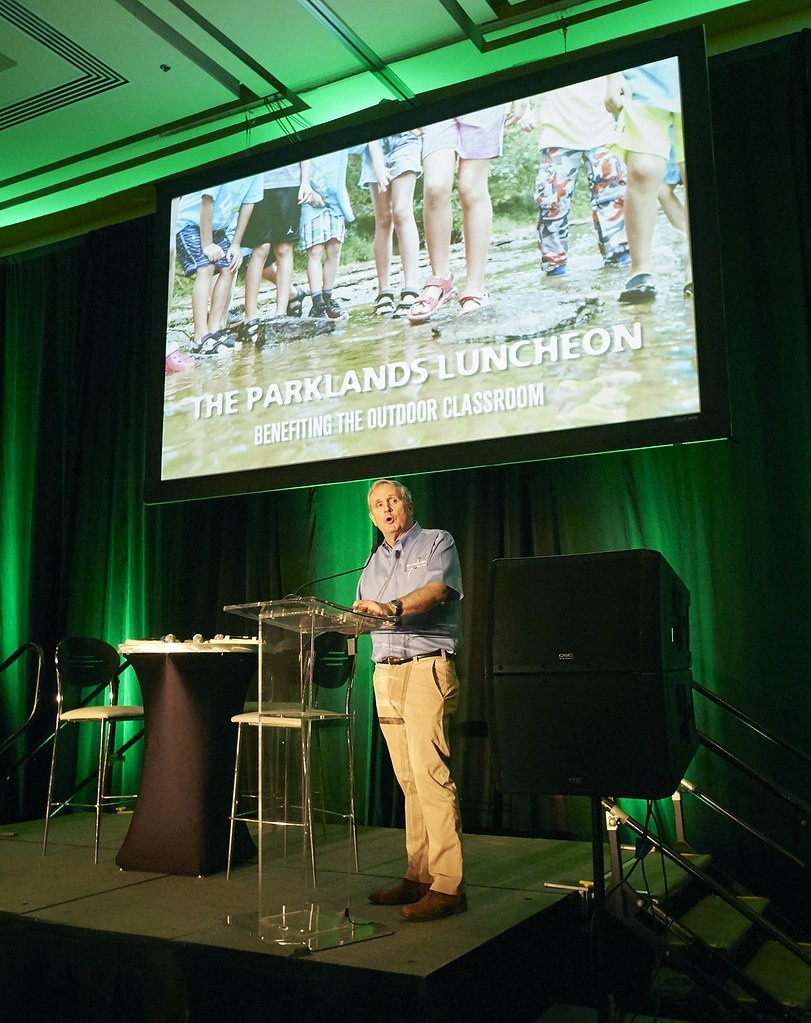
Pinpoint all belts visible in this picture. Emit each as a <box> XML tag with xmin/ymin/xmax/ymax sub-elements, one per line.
<box><xmin>379</xmin><ymin>648</ymin><xmax>455</xmax><ymax>665</ymax></box>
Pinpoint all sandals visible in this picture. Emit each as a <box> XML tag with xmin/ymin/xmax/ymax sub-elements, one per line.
<box><xmin>373</xmin><ymin>290</ymin><xmax>396</xmax><ymax>317</ymax></box>
<box><xmin>392</xmin><ymin>286</ymin><xmax>420</xmax><ymax>318</ymax></box>
<box><xmin>189</xmin><ymin>335</ymin><xmax>223</xmax><ymax>355</ymax></box>
<box><xmin>458</xmin><ymin>290</ymin><xmax>491</xmax><ymax>318</ymax></box>
<box><xmin>213</xmin><ymin>329</ymin><xmax>238</xmax><ymax>348</ymax></box>
<box><xmin>288</xmin><ymin>289</ymin><xmax>307</xmax><ymax>317</ymax></box>
<box><xmin>237</xmin><ymin>318</ymin><xmax>262</xmax><ymax>344</ymax></box>
<box><xmin>408</xmin><ymin>269</ymin><xmax>457</xmax><ymax>322</ymax></box>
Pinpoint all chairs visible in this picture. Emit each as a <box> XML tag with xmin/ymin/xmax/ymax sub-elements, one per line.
<box><xmin>226</xmin><ymin>630</ymin><xmax>359</xmax><ymax>888</ymax></box>
<box><xmin>233</xmin><ymin>642</ymin><xmax>328</xmax><ymax>845</ymax></box>
<box><xmin>42</xmin><ymin>637</ymin><xmax>145</xmax><ymax>865</ymax></box>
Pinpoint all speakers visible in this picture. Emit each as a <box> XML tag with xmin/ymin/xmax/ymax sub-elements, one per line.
<box><xmin>484</xmin><ymin>548</ymin><xmax>700</xmax><ymax>799</ymax></box>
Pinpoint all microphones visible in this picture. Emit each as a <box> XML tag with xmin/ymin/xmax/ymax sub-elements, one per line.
<box><xmin>395</xmin><ymin>550</ymin><xmax>400</xmax><ymax>559</ymax></box>
<box><xmin>283</xmin><ymin>544</ymin><xmax>379</xmax><ymax>600</ymax></box>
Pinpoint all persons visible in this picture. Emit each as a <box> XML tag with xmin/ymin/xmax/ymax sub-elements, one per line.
<box><xmin>407</xmin><ymin>99</ymin><xmax>524</xmax><ymax>323</ymax></box>
<box><xmin>300</xmin><ymin>137</ymin><xmax>397</xmax><ymax>319</ymax></box>
<box><xmin>176</xmin><ymin>173</ymin><xmax>264</xmax><ymax>356</ymax></box>
<box><xmin>517</xmin><ymin>71</ymin><xmax>633</xmax><ymax>277</ymax></box>
<box><xmin>353</xmin><ymin>480</ymin><xmax>469</xmax><ymax>921</ymax></box>
<box><xmin>604</xmin><ymin>57</ymin><xmax>695</xmax><ymax>302</ymax></box>
<box><xmin>356</xmin><ymin>128</ymin><xmax>423</xmax><ymax>316</ymax></box>
<box><xmin>213</xmin><ymin>158</ymin><xmax>313</xmax><ymax>343</ymax></box>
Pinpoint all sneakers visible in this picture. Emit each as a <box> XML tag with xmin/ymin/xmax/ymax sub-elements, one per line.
<box><xmin>369</xmin><ymin>879</ymin><xmax>433</xmax><ymax>904</ymax></box>
<box><xmin>400</xmin><ymin>887</ymin><xmax>468</xmax><ymax>922</ymax></box>
<box><xmin>312</xmin><ymin>300</ymin><xmax>348</xmax><ymax>323</ymax></box>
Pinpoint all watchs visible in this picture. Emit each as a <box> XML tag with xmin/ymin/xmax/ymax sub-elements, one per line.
<box><xmin>389</xmin><ymin>599</ymin><xmax>404</xmax><ymax>617</ymax></box>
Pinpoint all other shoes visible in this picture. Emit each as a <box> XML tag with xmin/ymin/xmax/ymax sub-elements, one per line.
<box><xmin>621</xmin><ymin>275</ymin><xmax>657</xmax><ymax>301</ymax></box>
<box><xmin>684</xmin><ymin>275</ymin><xmax>693</xmax><ymax>295</ymax></box>
<box><xmin>166</xmin><ymin>341</ymin><xmax>191</xmax><ymax>373</ymax></box>
